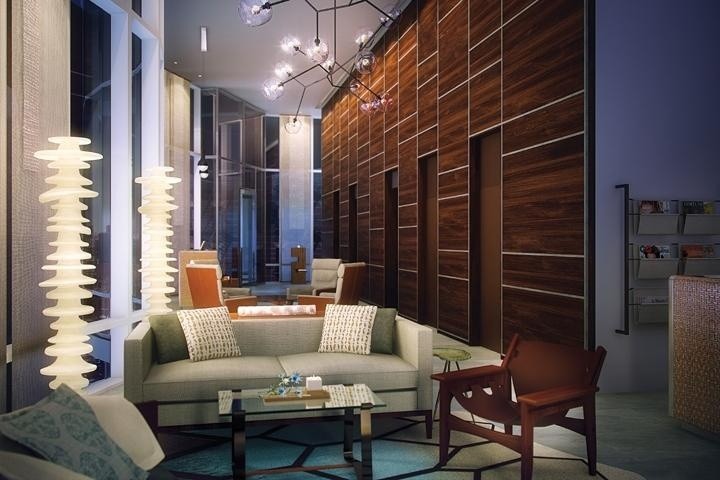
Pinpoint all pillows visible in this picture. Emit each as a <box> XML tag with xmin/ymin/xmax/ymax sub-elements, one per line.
<box><xmin>318</xmin><ymin>303</ymin><xmax>377</xmax><ymax>355</ymax></box>
<box><xmin>177</xmin><ymin>306</ymin><xmax>241</xmax><ymax>363</ymax></box>
<box><xmin>358</xmin><ymin>300</ymin><xmax>396</xmax><ymax>354</ymax></box>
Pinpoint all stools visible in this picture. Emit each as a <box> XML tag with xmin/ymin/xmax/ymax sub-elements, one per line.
<box><xmin>432</xmin><ymin>348</ymin><xmax>475</xmax><ymax>425</ymax></box>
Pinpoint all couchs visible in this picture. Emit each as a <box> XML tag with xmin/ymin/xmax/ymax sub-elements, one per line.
<box><xmin>124</xmin><ymin>315</ymin><xmax>437</xmax><ymax>438</ymax></box>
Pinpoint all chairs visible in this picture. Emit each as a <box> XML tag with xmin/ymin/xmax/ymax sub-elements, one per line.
<box><xmin>179</xmin><ymin>251</ymin><xmax>366</xmax><ymax>312</ymax></box>
<box><xmin>431</xmin><ymin>332</ymin><xmax>607</xmax><ymax>479</ymax></box>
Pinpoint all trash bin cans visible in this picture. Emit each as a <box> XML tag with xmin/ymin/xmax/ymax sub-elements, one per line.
<box><xmin>291</xmin><ymin>244</ymin><xmax>307</xmax><ymax>284</ymax></box>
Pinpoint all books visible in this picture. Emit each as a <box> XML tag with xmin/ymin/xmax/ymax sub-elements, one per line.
<box><xmin>259</xmin><ymin>388</ymin><xmax>332</xmax><ymax>406</ymax></box>
<box><xmin>634</xmin><ymin>195</ymin><xmax>719</xmax><ymax>307</ymax></box>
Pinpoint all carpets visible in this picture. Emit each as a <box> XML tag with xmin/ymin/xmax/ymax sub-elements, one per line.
<box><xmin>158</xmin><ymin>410</ymin><xmax>650</xmax><ymax>478</ymax></box>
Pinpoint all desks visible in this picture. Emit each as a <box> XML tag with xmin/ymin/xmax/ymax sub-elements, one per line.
<box><xmin>230</xmin><ymin>311</ymin><xmax>325</xmax><ymax>320</ymax></box>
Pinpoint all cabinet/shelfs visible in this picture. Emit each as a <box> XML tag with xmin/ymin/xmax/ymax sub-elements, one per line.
<box><xmin>615</xmin><ymin>184</ymin><xmax>720</xmax><ymax>335</ymax></box>
<box><xmin>668</xmin><ymin>274</ymin><xmax>720</xmax><ymax>444</ymax></box>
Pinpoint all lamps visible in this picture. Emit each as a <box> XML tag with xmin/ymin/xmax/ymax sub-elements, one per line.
<box><xmin>199</xmin><ymin>170</ymin><xmax>209</xmax><ymax>179</ymax></box>
<box><xmin>189</xmin><ymin>151</ymin><xmax>209</xmax><ymax>171</ymax></box>
<box><xmin>201</xmin><ymin>27</ymin><xmax>207</xmax><ymax>52</ymax></box>
<box><xmin>238</xmin><ymin>0</ymin><xmax>401</xmax><ymax>134</ymax></box>
